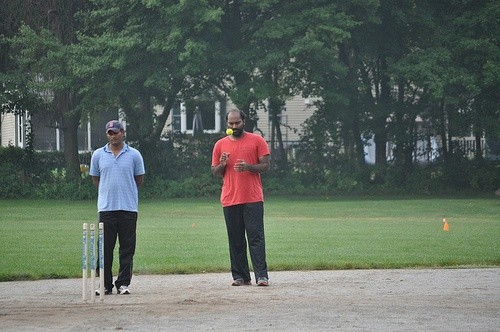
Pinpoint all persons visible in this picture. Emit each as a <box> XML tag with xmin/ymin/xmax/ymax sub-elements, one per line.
<box><xmin>211</xmin><ymin>109</ymin><xmax>271</xmax><ymax>286</ymax></box>
<box><xmin>89</xmin><ymin>120</ymin><xmax>145</xmax><ymax>296</ymax></box>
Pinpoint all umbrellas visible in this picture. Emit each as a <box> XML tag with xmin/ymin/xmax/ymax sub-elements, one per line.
<box><xmin>192</xmin><ymin>106</ymin><xmax>205</xmax><ymax>136</ymax></box>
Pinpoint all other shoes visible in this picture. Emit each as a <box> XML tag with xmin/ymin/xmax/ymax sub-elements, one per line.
<box><xmin>116</xmin><ymin>285</ymin><xmax>131</xmax><ymax>294</ymax></box>
<box><xmin>94</xmin><ymin>286</ymin><xmax>112</xmax><ymax>295</ymax></box>
<box><xmin>231</xmin><ymin>277</ymin><xmax>252</xmax><ymax>286</ymax></box>
<box><xmin>256</xmin><ymin>278</ymin><xmax>269</xmax><ymax>286</ymax></box>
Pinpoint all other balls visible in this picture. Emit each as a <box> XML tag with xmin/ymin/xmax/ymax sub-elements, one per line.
<box><xmin>226</xmin><ymin>128</ymin><xmax>233</xmax><ymax>136</ymax></box>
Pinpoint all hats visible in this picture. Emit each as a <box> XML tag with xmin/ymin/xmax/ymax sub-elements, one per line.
<box><xmin>105</xmin><ymin>119</ymin><xmax>124</xmax><ymax>134</ymax></box>
